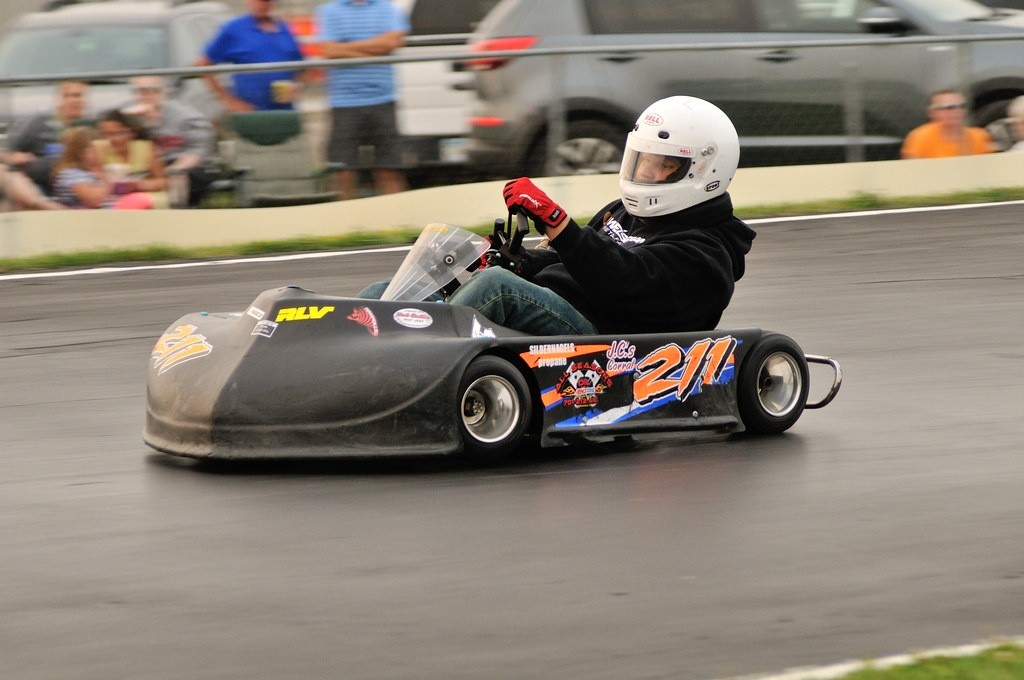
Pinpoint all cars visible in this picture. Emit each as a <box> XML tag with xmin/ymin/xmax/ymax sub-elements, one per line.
<box><xmin>391</xmin><ymin>0</ymin><xmax>1024</xmax><ymax>179</ymax></box>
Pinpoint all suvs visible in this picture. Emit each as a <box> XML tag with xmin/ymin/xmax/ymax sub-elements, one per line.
<box><xmin>0</xmin><ymin>2</ymin><xmax>233</xmax><ymax>137</ymax></box>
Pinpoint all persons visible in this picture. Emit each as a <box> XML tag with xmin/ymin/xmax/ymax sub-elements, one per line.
<box><xmin>0</xmin><ymin>75</ymin><xmax>213</xmax><ymax>211</ymax></box>
<box><xmin>355</xmin><ymin>94</ymin><xmax>757</xmax><ymax>335</ymax></box>
<box><xmin>313</xmin><ymin>1</ymin><xmax>409</xmax><ymax>200</ymax></box>
<box><xmin>195</xmin><ymin>0</ymin><xmax>303</xmax><ymax>112</ymax></box>
<box><xmin>1002</xmin><ymin>94</ymin><xmax>1023</xmax><ymax>153</ymax></box>
<box><xmin>899</xmin><ymin>90</ymin><xmax>997</xmax><ymax>159</ymax></box>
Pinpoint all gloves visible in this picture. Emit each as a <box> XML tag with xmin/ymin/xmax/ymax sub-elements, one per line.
<box><xmin>504</xmin><ymin>178</ymin><xmax>567</xmax><ymax>227</ymax></box>
<box><xmin>475</xmin><ymin>235</ymin><xmax>497</xmax><ymax>270</ymax></box>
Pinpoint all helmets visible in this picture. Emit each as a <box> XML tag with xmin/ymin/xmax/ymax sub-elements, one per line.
<box><xmin>619</xmin><ymin>94</ymin><xmax>740</xmax><ymax>217</ymax></box>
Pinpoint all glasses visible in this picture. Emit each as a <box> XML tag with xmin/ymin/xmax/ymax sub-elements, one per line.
<box><xmin>932</xmin><ymin>99</ymin><xmax>968</xmax><ymax>113</ymax></box>
